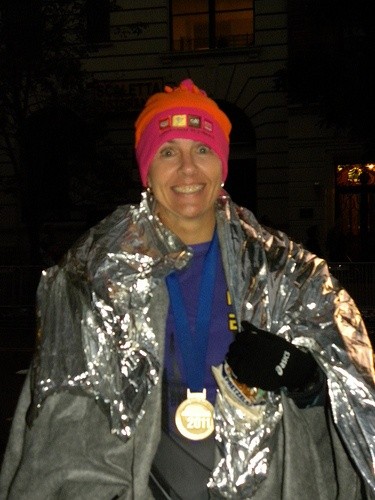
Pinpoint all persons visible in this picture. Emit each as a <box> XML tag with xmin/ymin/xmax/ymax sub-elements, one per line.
<box><xmin>6</xmin><ymin>76</ymin><xmax>375</xmax><ymax>499</ymax></box>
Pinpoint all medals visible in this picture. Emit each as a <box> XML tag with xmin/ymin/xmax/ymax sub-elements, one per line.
<box><xmin>175</xmin><ymin>388</ymin><xmax>216</xmax><ymax>440</ymax></box>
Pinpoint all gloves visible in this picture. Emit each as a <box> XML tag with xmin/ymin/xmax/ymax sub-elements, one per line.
<box><xmin>228</xmin><ymin>321</ymin><xmax>317</xmax><ymax>394</ymax></box>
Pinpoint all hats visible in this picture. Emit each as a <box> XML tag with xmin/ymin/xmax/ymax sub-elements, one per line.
<box><xmin>135</xmin><ymin>79</ymin><xmax>232</xmax><ymax>186</ymax></box>
